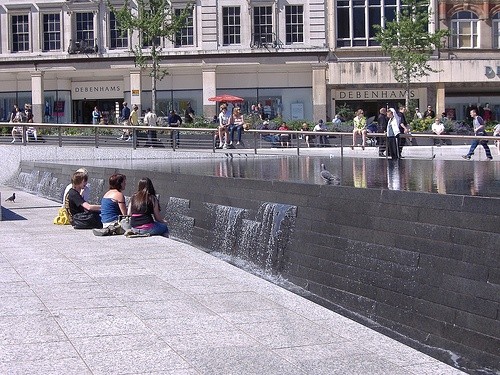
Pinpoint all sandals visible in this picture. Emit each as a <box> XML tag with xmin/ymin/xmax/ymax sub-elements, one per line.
<box><xmin>126</xmin><ymin>231</ymin><xmax>150</xmax><ymax>237</ymax></box>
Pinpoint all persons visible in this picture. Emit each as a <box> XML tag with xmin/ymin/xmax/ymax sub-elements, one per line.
<box><xmin>9</xmin><ymin>102</ymin><xmax>500</xmax><ymax>163</ymax></box>
<box><xmin>63</xmin><ymin>167</ymin><xmax>170</xmax><ymax>238</ymax></box>
<box><xmin>465</xmin><ymin>103</ymin><xmax>474</xmax><ymax>125</ymax></box>
<box><xmin>483</xmin><ymin>103</ymin><xmax>490</xmax><ymax>123</ymax></box>
<box><xmin>475</xmin><ymin>102</ymin><xmax>484</xmax><ymax>118</ymax></box>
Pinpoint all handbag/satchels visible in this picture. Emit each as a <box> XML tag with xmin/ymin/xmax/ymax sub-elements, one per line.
<box><xmin>53</xmin><ymin>206</ymin><xmax>72</xmax><ymax>224</ymax></box>
<box><xmin>482</xmin><ymin>130</ymin><xmax>489</xmax><ymax>143</ymax></box>
<box><xmin>73</xmin><ymin>212</ymin><xmax>103</xmax><ymax>229</ymax></box>
<box><xmin>281</xmin><ymin>133</ymin><xmax>289</xmax><ymax>136</ymax></box>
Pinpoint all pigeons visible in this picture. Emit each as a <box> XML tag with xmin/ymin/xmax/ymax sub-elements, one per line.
<box><xmin>4</xmin><ymin>192</ymin><xmax>16</xmax><ymax>202</ymax></box>
<box><xmin>319</xmin><ymin>163</ymin><xmax>340</xmax><ymax>183</ymax></box>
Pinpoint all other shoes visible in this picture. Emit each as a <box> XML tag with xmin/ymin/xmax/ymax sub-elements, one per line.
<box><xmin>219</xmin><ymin>142</ymin><xmax>223</xmax><ymax>148</ymax></box>
<box><xmin>399</xmin><ymin>153</ymin><xmax>405</xmax><ymax>158</ymax></box>
<box><xmin>230</xmin><ymin>140</ymin><xmax>233</xmax><ymax>145</ymax></box>
<box><xmin>462</xmin><ymin>155</ymin><xmax>470</xmax><ymax>160</ymax></box>
<box><xmin>117</xmin><ymin>134</ymin><xmax>123</xmax><ymax>140</ymax></box>
<box><xmin>223</xmin><ymin>141</ymin><xmax>227</xmax><ymax>147</ymax></box>
<box><xmin>125</xmin><ymin>136</ymin><xmax>132</xmax><ymax>141</ymax></box>
<box><xmin>379</xmin><ymin>152</ymin><xmax>386</xmax><ymax>157</ymax></box>
<box><xmin>485</xmin><ymin>158</ymin><xmax>494</xmax><ymax>161</ymax></box>
<box><xmin>236</xmin><ymin>141</ymin><xmax>242</xmax><ymax>145</ymax></box>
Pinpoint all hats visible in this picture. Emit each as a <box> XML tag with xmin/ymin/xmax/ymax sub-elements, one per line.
<box><xmin>319</xmin><ymin>120</ymin><xmax>323</xmax><ymax>123</ymax></box>
<box><xmin>123</xmin><ymin>102</ymin><xmax>127</xmax><ymax>105</ymax></box>
<box><xmin>263</xmin><ymin>121</ymin><xmax>269</xmax><ymax>125</ymax></box>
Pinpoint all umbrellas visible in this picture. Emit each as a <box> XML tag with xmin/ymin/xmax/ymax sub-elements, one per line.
<box><xmin>208</xmin><ymin>95</ymin><xmax>244</xmax><ymax>102</ymax></box>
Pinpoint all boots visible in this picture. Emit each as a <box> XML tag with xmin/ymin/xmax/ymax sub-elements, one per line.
<box><xmin>93</xmin><ymin>222</ymin><xmax>126</xmax><ymax>236</ymax></box>
